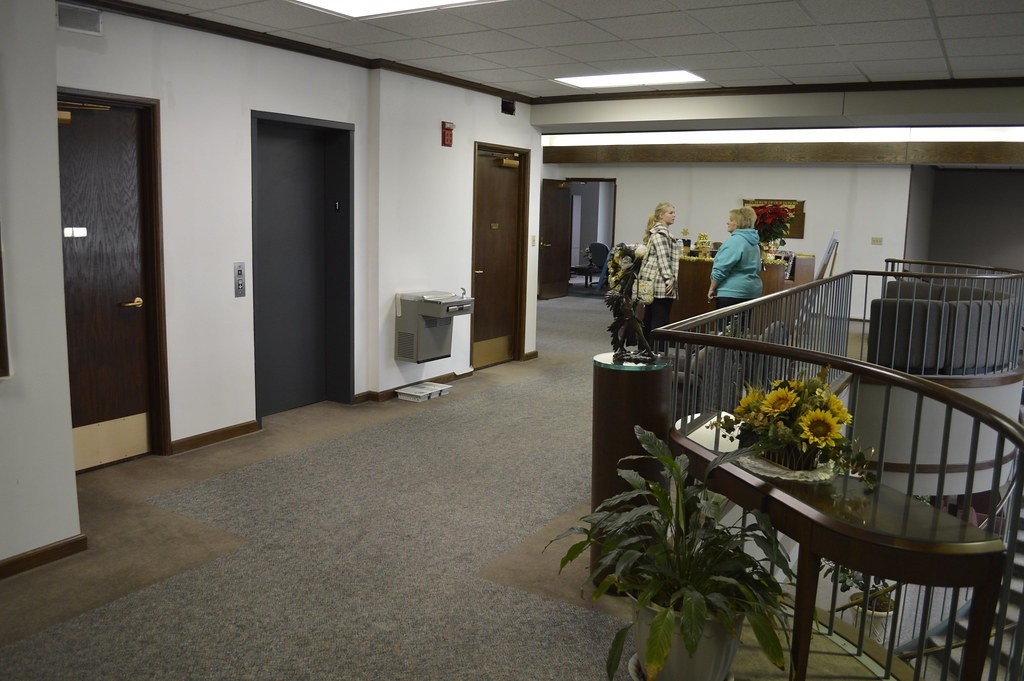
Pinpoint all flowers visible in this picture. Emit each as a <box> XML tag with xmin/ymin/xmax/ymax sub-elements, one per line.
<box><xmin>705</xmin><ymin>363</ymin><xmax>878</xmax><ymax>495</ymax></box>
<box><xmin>583</xmin><ymin>247</ymin><xmax>595</xmax><ymax>261</ymax></box>
<box><xmin>750</xmin><ymin>203</ymin><xmax>799</xmax><ymax>246</ymax></box>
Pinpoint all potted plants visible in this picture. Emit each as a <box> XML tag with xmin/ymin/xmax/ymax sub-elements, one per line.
<box><xmin>541</xmin><ymin>425</ymin><xmax>820</xmax><ymax>681</ymax></box>
<box><xmin>818</xmin><ymin>553</ymin><xmax>897</xmax><ymax>645</ymax></box>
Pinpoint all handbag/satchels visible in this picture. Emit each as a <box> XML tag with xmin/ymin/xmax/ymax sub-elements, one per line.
<box><xmin>631</xmin><ymin>278</ymin><xmax>656</xmax><ymax>306</ymax></box>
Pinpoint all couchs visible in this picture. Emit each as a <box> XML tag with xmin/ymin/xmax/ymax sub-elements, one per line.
<box><xmin>663</xmin><ymin>330</ymin><xmax>735</xmax><ymax>414</ymax></box>
<box><xmin>741</xmin><ymin>319</ymin><xmax>787</xmax><ymax>387</ymax></box>
<box><xmin>867</xmin><ymin>280</ymin><xmax>1019</xmax><ymax>374</ymax></box>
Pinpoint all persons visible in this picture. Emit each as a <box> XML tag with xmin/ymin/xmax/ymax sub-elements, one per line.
<box><xmin>707</xmin><ymin>206</ymin><xmax>763</xmax><ymax>330</ymax></box>
<box><xmin>631</xmin><ymin>202</ymin><xmax>679</xmax><ymax>351</ymax></box>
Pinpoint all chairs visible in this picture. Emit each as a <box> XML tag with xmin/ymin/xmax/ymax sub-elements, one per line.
<box><xmin>588</xmin><ymin>242</ymin><xmax>612</xmax><ymax>287</ymax></box>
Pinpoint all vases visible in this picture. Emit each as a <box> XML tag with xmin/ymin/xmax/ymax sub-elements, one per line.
<box><xmin>760</xmin><ymin>236</ymin><xmax>780</xmax><ymax>253</ymax></box>
<box><xmin>738</xmin><ymin>429</ymin><xmax>822</xmax><ymax>471</ymax></box>
<box><xmin>588</xmin><ymin>260</ymin><xmax>593</xmax><ymax>265</ymax></box>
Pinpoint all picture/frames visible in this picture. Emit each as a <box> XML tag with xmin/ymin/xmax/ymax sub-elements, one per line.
<box><xmin>743</xmin><ymin>198</ymin><xmax>805</xmax><ymax>238</ymax></box>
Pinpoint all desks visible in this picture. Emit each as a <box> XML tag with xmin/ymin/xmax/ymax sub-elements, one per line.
<box><xmin>569</xmin><ymin>263</ymin><xmax>602</xmax><ymax>287</ymax></box>
